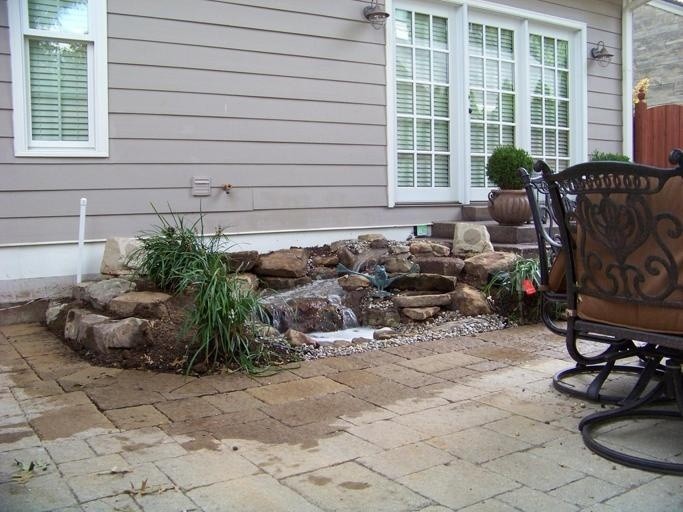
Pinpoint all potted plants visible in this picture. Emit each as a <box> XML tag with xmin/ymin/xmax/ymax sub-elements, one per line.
<box><xmin>485</xmin><ymin>144</ymin><xmax>533</xmax><ymax>227</ymax></box>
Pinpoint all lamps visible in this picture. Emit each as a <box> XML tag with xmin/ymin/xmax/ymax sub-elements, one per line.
<box><xmin>591</xmin><ymin>41</ymin><xmax>614</xmax><ymax>67</ymax></box>
<box><xmin>362</xmin><ymin>0</ymin><xmax>390</xmax><ymax>30</ymax></box>
